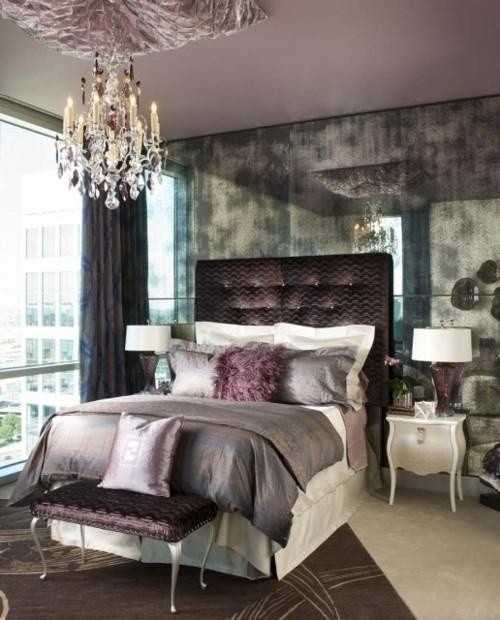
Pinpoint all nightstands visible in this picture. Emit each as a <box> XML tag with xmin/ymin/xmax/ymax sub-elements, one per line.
<box><xmin>384</xmin><ymin>411</ymin><xmax>467</xmax><ymax>512</ymax></box>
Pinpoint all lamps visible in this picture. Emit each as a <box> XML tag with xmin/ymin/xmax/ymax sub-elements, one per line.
<box><xmin>352</xmin><ymin>197</ymin><xmax>398</xmax><ymax>262</ymax></box>
<box><xmin>54</xmin><ymin>46</ymin><xmax>169</xmax><ymax>211</ymax></box>
<box><xmin>124</xmin><ymin>323</ymin><xmax>171</xmax><ymax>394</ymax></box>
<box><xmin>410</xmin><ymin>328</ymin><xmax>472</xmax><ymax>417</ymax></box>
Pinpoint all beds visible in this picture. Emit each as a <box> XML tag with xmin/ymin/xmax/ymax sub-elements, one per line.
<box><xmin>6</xmin><ymin>254</ymin><xmax>394</xmax><ymax>576</ymax></box>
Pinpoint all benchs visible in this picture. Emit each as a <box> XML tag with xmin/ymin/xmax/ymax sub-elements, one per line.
<box><xmin>28</xmin><ymin>479</ymin><xmax>221</xmax><ymax>614</ymax></box>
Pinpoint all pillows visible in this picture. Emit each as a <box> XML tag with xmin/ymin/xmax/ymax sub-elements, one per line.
<box><xmin>166</xmin><ymin>321</ymin><xmax>375</xmax><ymax>412</ymax></box>
<box><xmin>97</xmin><ymin>411</ymin><xmax>185</xmax><ymax>498</ymax></box>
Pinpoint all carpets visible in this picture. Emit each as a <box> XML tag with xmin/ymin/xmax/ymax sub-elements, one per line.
<box><xmin>2</xmin><ymin>498</ymin><xmax>416</xmax><ymax>620</ymax></box>
